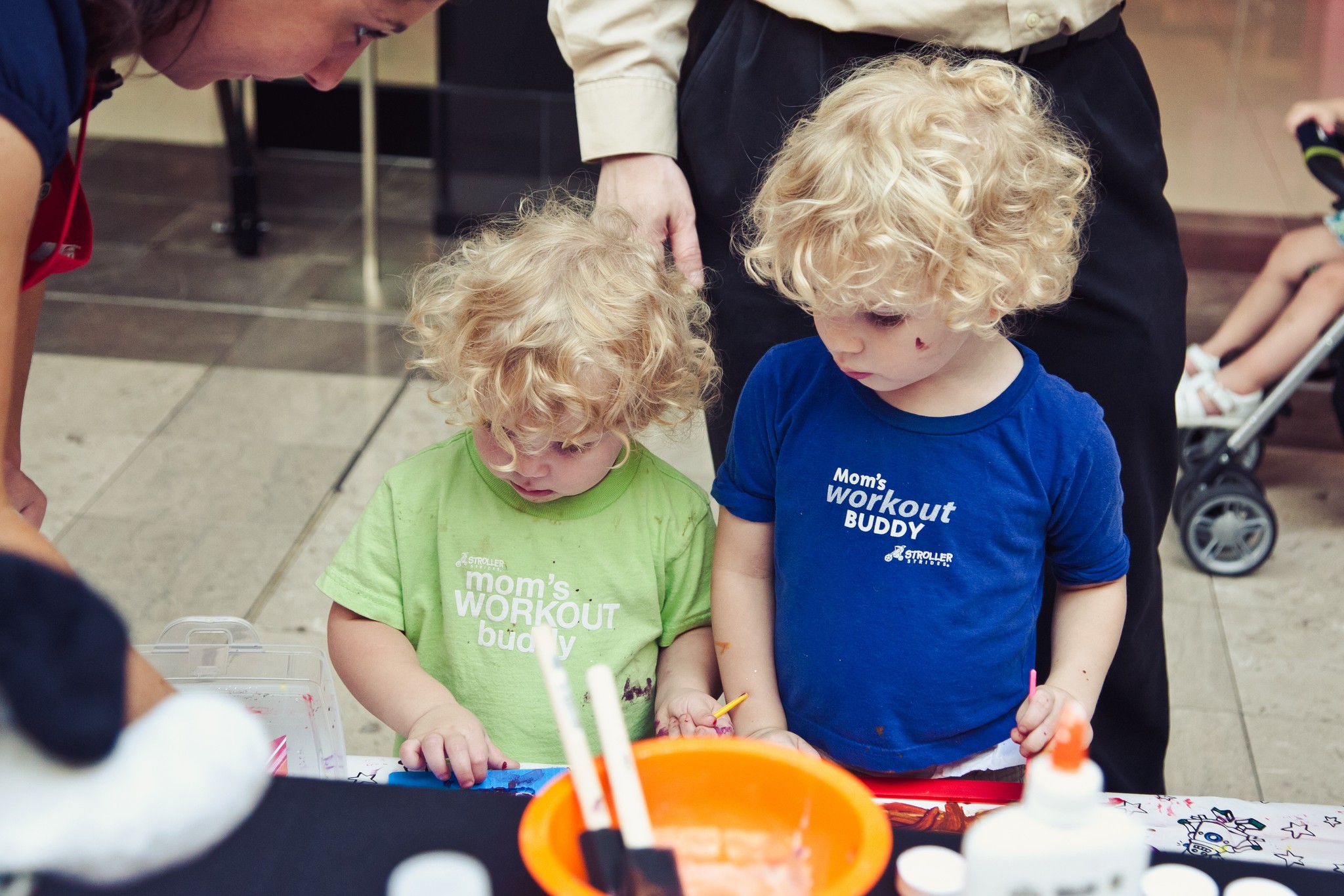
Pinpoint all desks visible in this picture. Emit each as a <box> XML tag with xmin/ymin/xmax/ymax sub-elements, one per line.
<box><xmin>0</xmin><ymin>758</ymin><xmax>1344</xmax><ymax>896</ymax></box>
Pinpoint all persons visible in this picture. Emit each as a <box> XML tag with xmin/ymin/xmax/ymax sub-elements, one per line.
<box><xmin>0</xmin><ymin>0</ymin><xmax>449</xmax><ymax>723</ymax></box>
<box><xmin>315</xmin><ymin>187</ymin><xmax>733</xmax><ymax>789</ymax></box>
<box><xmin>710</xmin><ymin>53</ymin><xmax>1130</xmax><ymax>778</ymax></box>
<box><xmin>1174</xmin><ymin>100</ymin><xmax>1344</xmax><ymax>431</ymax></box>
<box><xmin>548</xmin><ymin>0</ymin><xmax>1187</xmax><ymax>792</ymax></box>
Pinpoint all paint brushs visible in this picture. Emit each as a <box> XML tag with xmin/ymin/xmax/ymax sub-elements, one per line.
<box><xmin>1023</xmin><ymin>668</ymin><xmax>1036</xmax><ymax>799</ymax></box>
<box><xmin>711</xmin><ymin>692</ymin><xmax>748</xmax><ymax>722</ymax></box>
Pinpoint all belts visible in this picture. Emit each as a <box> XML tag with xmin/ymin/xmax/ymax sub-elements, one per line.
<box><xmin>1003</xmin><ymin>2</ymin><xmax>1123</xmax><ymax>62</ymax></box>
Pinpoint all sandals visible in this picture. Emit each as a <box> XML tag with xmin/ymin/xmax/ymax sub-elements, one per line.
<box><xmin>1174</xmin><ymin>343</ymin><xmax>1222</xmax><ymax>385</ymax></box>
<box><xmin>1175</xmin><ymin>369</ymin><xmax>1262</xmax><ymax>431</ymax></box>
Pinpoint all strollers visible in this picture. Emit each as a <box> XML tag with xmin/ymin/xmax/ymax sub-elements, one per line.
<box><xmin>1169</xmin><ymin>117</ymin><xmax>1344</xmax><ymax>578</ymax></box>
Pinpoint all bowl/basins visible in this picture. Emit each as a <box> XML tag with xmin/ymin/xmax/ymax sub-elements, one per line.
<box><xmin>518</xmin><ymin>734</ymin><xmax>894</xmax><ymax>896</ymax></box>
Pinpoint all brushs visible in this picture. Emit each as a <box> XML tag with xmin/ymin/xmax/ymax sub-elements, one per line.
<box><xmin>539</xmin><ymin>623</ymin><xmax>624</xmax><ymax>896</ymax></box>
<box><xmin>586</xmin><ymin>664</ymin><xmax>679</xmax><ymax>896</ymax></box>
